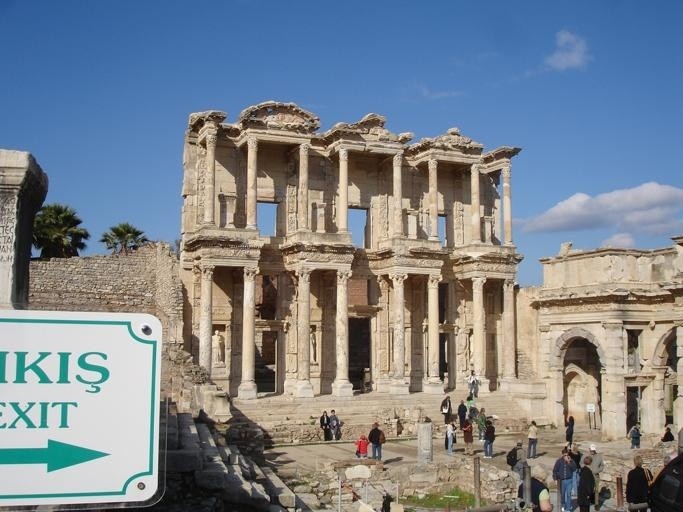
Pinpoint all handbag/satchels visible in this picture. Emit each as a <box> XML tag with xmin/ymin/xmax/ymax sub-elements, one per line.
<box><xmin>378</xmin><ymin>431</ymin><xmax>384</xmax><ymax>444</ymax></box>
<box><xmin>643</xmin><ymin>468</ymin><xmax>651</xmax><ymax>487</ymax></box>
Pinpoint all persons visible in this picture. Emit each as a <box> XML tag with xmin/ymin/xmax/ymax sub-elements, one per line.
<box><xmin>321</xmin><ymin>411</ymin><xmax>331</xmax><ymax>441</ymax></box>
<box><xmin>309</xmin><ymin>328</ymin><xmax>316</xmax><ymax>362</ymax></box>
<box><xmin>369</xmin><ymin>423</ymin><xmax>382</xmax><ymax>460</ymax></box>
<box><xmin>211</xmin><ymin>330</ymin><xmax>223</xmax><ymax>364</ymax></box>
<box><xmin>355</xmin><ymin>435</ymin><xmax>369</xmax><ymax>458</ymax></box>
<box><xmin>329</xmin><ymin>410</ymin><xmax>340</xmax><ymax>440</ymax></box>
<box><xmin>441</xmin><ymin>370</ymin><xmax>683</xmax><ymax>512</ymax></box>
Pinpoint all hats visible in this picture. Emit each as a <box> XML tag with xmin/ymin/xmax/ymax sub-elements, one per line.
<box><xmin>588</xmin><ymin>444</ymin><xmax>596</xmax><ymax>451</ymax></box>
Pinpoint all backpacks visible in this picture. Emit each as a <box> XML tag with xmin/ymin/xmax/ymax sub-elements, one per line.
<box><xmin>646</xmin><ymin>454</ymin><xmax>682</xmax><ymax>512</ymax></box>
<box><xmin>506</xmin><ymin>447</ymin><xmax>522</xmax><ymax>466</ymax></box>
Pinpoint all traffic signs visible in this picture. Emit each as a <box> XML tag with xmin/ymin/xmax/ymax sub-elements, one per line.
<box><xmin>0</xmin><ymin>313</ymin><xmax>161</xmax><ymax>506</ymax></box>
